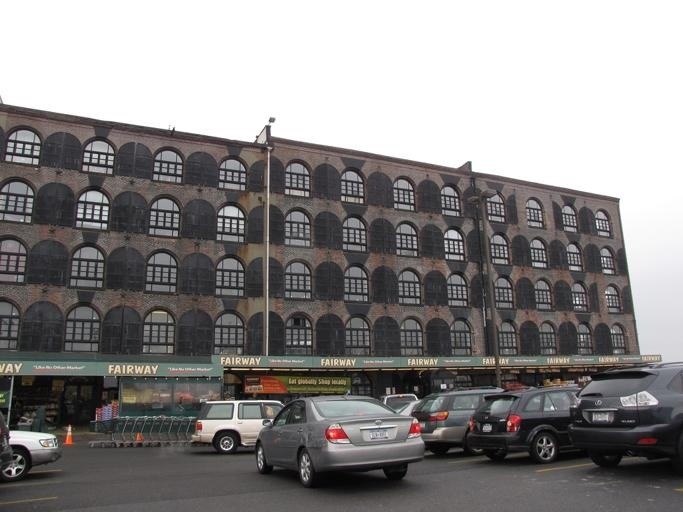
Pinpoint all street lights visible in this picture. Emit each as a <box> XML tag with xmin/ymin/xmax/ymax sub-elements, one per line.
<box><xmin>467</xmin><ymin>189</ymin><xmax>502</xmax><ymax>388</ymax></box>
<box><xmin>256</xmin><ymin>117</ymin><xmax>275</xmax><ymax>356</ymax></box>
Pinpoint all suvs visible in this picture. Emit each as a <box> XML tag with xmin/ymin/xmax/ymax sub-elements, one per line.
<box><xmin>411</xmin><ymin>387</ymin><xmax>505</xmax><ymax>456</ymax></box>
<box><xmin>465</xmin><ymin>385</ymin><xmax>582</xmax><ymax>464</ymax></box>
<box><xmin>567</xmin><ymin>360</ymin><xmax>683</xmax><ymax>470</ymax></box>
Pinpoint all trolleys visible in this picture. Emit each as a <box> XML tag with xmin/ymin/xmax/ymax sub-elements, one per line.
<box><xmin>89</xmin><ymin>415</ymin><xmax>192</xmax><ymax>448</ymax></box>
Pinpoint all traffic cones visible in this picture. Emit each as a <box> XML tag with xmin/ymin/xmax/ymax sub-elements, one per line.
<box><xmin>64</xmin><ymin>423</ymin><xmax>74</xmax><ymax>445</ymax></box>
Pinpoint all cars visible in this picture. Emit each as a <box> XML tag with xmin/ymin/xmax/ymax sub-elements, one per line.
<box><xmin>0</xmin><ymin>408</ymin><xmax>64</xmax><ymax>481</ymax></box>
<box><xmin>254</xmin><ymin>395</ymin><xmax>427</xmax><ymax>488</ymax></box>
<box><xmin>153</xmin><ymin>388</ymin><xmax>210</xmax><ymax>409</ymax></box>
<box><xmin>190</xmin><ymin>399</ymin><xmax>301</xmax><ymax>454</ymax></box>
<box><xmin>378</xmin><ymin>393</ymin><xmax>424</xmax><ymax>416</ymax></box>
